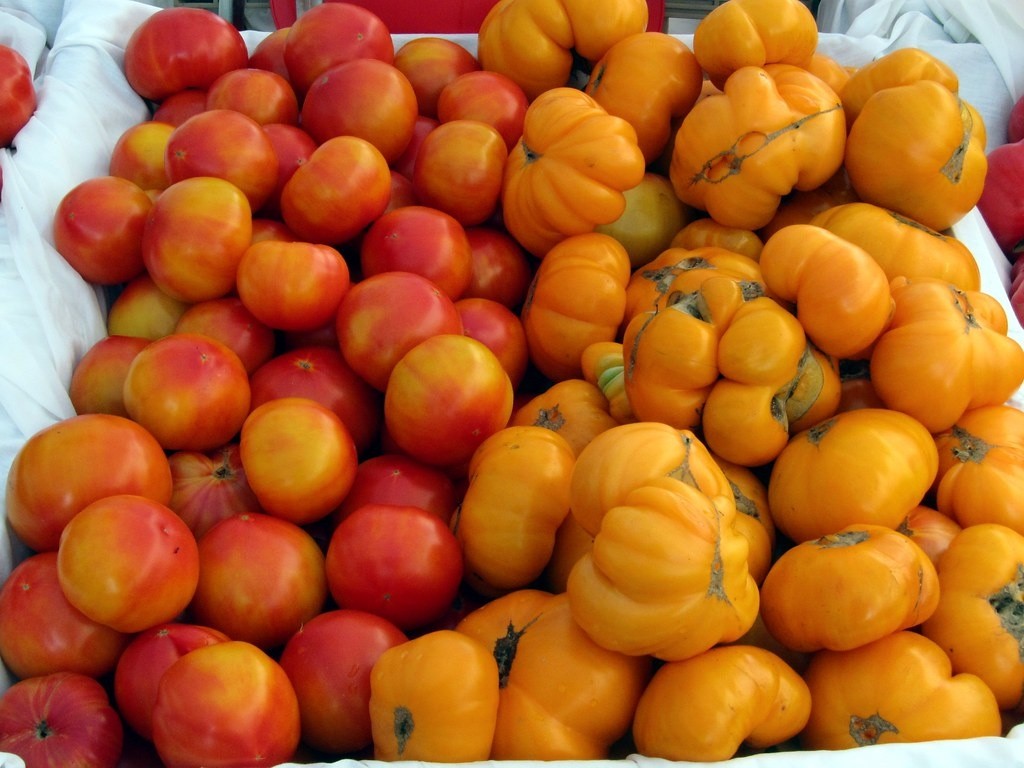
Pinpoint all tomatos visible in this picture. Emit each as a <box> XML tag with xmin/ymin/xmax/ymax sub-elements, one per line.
<box><xmin>0</xmin><ymin>0</ymin><xmax>1024</xmax><ymax>768</ymax></box>
